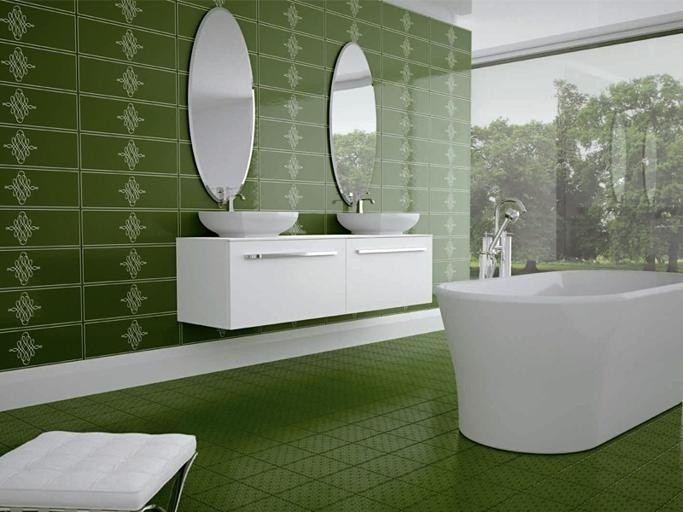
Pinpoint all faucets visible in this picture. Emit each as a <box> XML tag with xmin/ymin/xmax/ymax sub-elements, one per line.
<box><xmin>347</xmin><ymin>192</ymin><xmax>355</xmax><ymax>204</ymax></box>
<box><xmin>211</xmin><ymin>185</ymin><xmax>224</xmax><ymax>201</ymax></box>
<box><xmin>226</xmin><ymin>187</ymin><xmax>246</xmax><ymax>213</ymax></box>
<box><xmin>356</xmin><ymin>192</ymin><xmax>375</xmax><ymax>213</ymax></box>
<box><xmin>494</xmin><ymin>197</ymin><xmax>527</xmax><ymax>252</ymax></box>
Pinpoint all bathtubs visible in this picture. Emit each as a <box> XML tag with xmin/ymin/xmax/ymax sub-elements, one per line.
<box><xmin>435</xmin><ymin>268</ymin><xmax>683</xmax><ymax>454</ymax></box>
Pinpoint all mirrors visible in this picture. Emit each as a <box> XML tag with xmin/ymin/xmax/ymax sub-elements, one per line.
<box><xmin>187</xmin><ymin>4</ymin><xmax>256</xmax><ymax>205</ymax></box>
<box><xmin>328</xmin><ymin>41</ymin><xmax>378</xmax><ymax>209</ymax></box>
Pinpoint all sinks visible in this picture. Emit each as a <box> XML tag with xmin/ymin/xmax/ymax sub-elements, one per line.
<box><xmin>197</xmin><ymin>210</ymin><xmax>299</xmax><ymax>238</ymax></box>
<box><xmin>336</xmin><ymin>211</ymin><xmax>420</xmax><ymax>235</ymax></box>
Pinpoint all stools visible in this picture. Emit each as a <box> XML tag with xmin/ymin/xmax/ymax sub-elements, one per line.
<box><xmin>0</xmin><ymin>430</ymin><xmax>199</xmax><ymax>512</ymax></box>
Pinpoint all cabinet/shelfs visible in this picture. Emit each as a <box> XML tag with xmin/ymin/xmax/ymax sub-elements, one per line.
<box><xmin>176</xmin><ymin>237</ymin><xmax>433</xmax><ymax>331</ymax></box>
